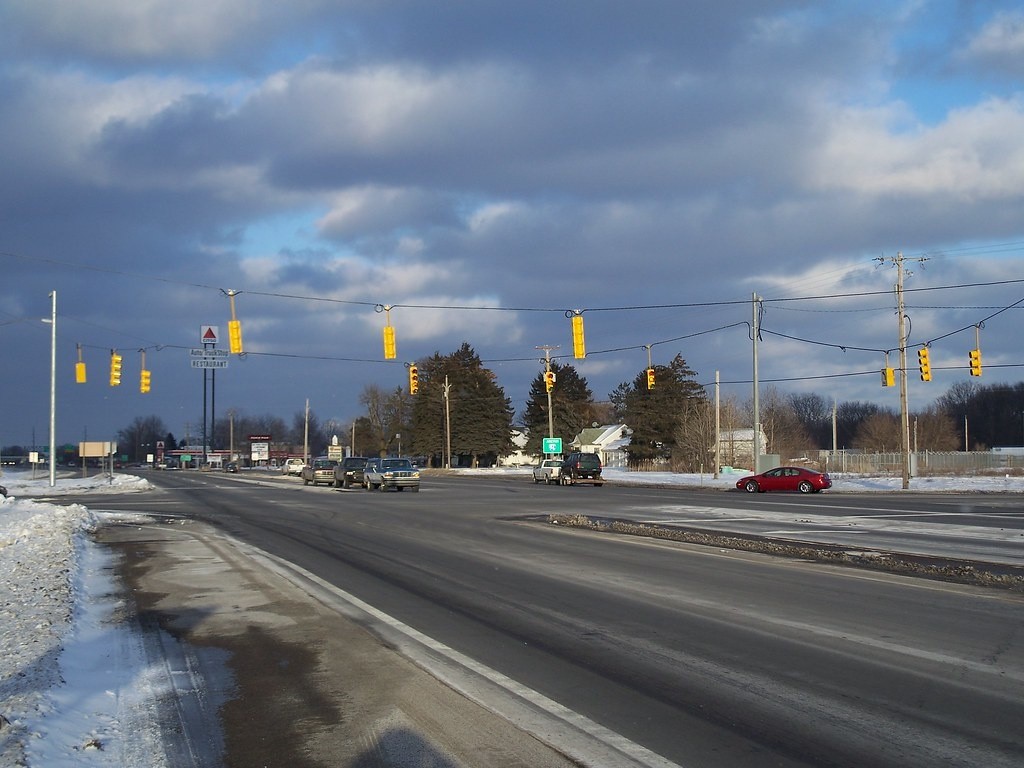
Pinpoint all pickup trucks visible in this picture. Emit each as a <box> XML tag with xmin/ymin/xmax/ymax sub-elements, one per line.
<box><xmin>333</xmin><ymin>457</ymin><xmax>369</xmax><ymax>489</ymax></box>
<box><xmin>301</xmin><ymin>457</ymin><xmax>338</xmax><ymax>486</ymax></box>
<box><xmin>532</xmin><ymin>459</ymin><xmax>566</xmax><ymax>484</ymax></box>
<box><xmin>281</xmin><ymin>458</ymin><xmax>307</xmax><ymax>476</ymax></box>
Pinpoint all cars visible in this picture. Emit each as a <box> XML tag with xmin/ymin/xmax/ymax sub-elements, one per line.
<box><xmin>66</xmin><ymin>458</ymin><xmax>141</xmax><ymax>468</ymax></box>
<box><xmin>226</xmin><ymin>463</ymin><xmax>238</xmax><ymax>473</ymax></box>
<box><xmin>736</xmin><ymin>465</ymin><xmax>830</xmax><ymax>493</ymax></box>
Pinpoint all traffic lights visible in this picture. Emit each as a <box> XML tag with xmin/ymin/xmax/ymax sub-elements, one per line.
<box><xmin>109</xmin><ymin>353</ymin><xmax>123</xmax><ymax>387</ymax></box>
<box><xmin>647</xmin><ymin>369</ymin><xmax>656</xmax><ymax>390</ymax></box>
<box><xmin>410</xmin><ymin>366</ymin><xmax>419</xmax><ymax>395</ymax></box>
<box><xmin>968</xmin><ymin>348</ymin><xmax>981</xmax><ymax>378</ymax></box>
<box><xmin>139</xmin><ymin>369</ymin><xmax>151</xmax><ymax>392</ymax></box>
<box><xmin>545</xmin><ymin>371</ymin><xmax>554</xmax><ymax>393</ymax></box>
<box><xmin>917</xmin><ymin>348</ymin><xmax>931</xmax><ymax>381</ymax></box>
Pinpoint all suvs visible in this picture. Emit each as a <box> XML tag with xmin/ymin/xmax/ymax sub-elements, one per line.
<box><xmin>562</xmin><ymin>451</ymin><xmax>603</xmax><ymax>479</ymax></box>
<box><xmin>363</xmin><ymin>457</ymin><xmax>420</xmax><ymax>493</ymax></box>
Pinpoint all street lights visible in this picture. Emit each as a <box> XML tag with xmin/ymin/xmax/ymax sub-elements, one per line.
<box><xmin>48</xmin><ymin>289</ymin><xmax>58</xmax><ymax>486</ymax></box>
